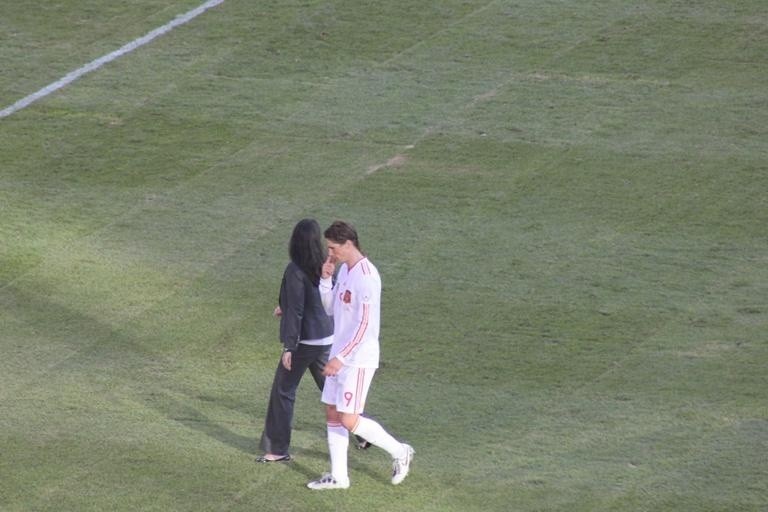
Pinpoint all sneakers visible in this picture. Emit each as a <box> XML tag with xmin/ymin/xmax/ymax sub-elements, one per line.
<box><xmin>306</xmin><ymin>475</ymin><xmax>350</xmax><ymax>493</ymax></box>
<box><xmin>390</xmin><ymin>442</ymin><xmax>417</xmax><ymax>487</ymax></box>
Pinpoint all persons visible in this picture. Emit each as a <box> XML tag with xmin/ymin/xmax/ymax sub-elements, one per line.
<box><xmin>256</xmin><ymin>217</ymin><xmax>377</xmax><ymax>462</ymax></box>
<box><xmin>305</xmin><ymin>222</ymin><xmax>415</xmax><ymax>490</ymax></box>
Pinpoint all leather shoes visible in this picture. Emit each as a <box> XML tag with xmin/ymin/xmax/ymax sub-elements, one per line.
<box><xmin>255</xmin><ymin>454</ymin><xmax>291</xmax><ymax>462</ymax></box>
